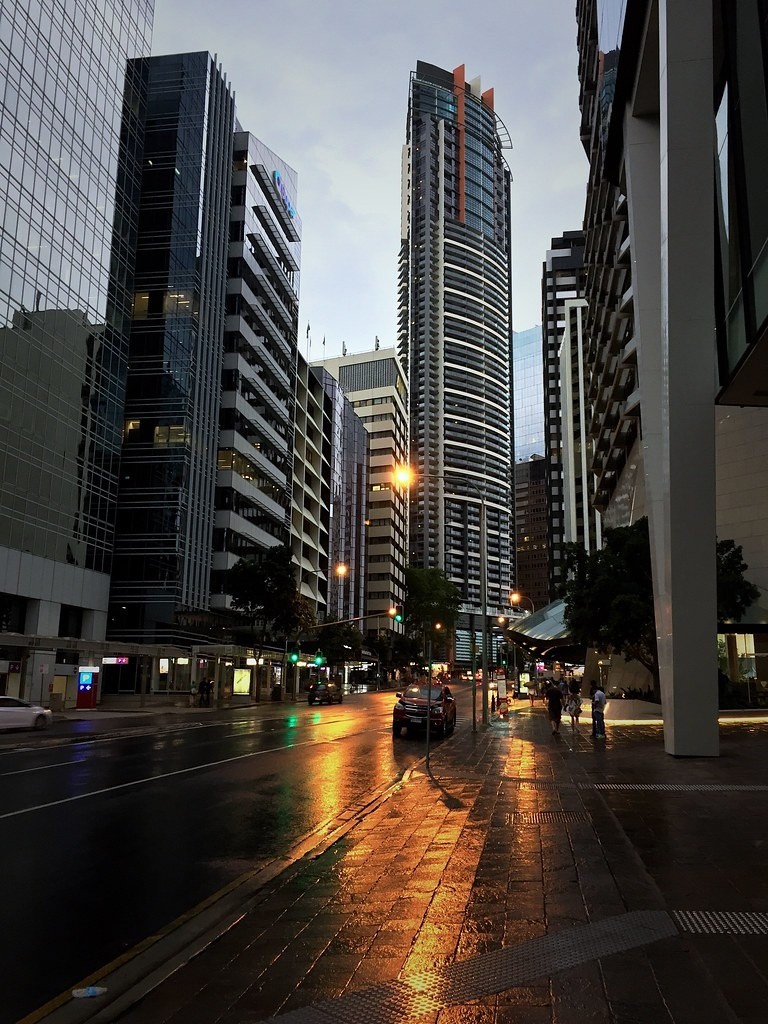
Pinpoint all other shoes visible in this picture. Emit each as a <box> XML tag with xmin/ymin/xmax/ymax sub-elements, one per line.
<box><xmin>552</xmin><ymin>730</ymin><xmax>560</xmax><ymax>735</ymax></box>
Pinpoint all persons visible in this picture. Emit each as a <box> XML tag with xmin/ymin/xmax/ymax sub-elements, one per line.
<box><xmin>189</xmin><ymin>676</ymin><xmax>214</xmax><ymax>706</ymax></box>
<box><xmin>526</xmin><ymin>673</ymin><xmax>607</xmax><ymax>736</ymax></box>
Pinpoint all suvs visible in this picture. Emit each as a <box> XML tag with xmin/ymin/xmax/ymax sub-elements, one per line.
<box><xmin>393</xmin><ymin>682</ymin><xmax>457</xmax><ymax>741</ymax></box>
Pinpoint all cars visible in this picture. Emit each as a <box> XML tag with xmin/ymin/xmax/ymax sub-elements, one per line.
<box><xmin>308</xmin><ymin>682</ymin><xmax>343</xmax><ymax>705</ymax></box>
<box><xmin>0</xmin><ymin>694</ymin><xmax>54</xmax><ymax>732</ymax></box>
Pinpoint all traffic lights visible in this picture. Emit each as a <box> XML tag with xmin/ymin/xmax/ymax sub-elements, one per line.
<box><xmin>501</xmin><ymin>653</ymin><xmax>507</xmax><ymax>665</ymax></box>
<box><xmin>314</xmin><ymin>651</ymin><xmax>322</xmax><ymax>665</ymax></box>
<box><xmin>394</xmin><ymin>605</ymin><xmax>403</xmax><ymax>623</ymax></box>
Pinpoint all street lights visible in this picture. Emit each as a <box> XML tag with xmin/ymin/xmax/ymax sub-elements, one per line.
<box><xmin>394</xmin><ymin>470</ymin><xmax>489</xmax><ymax>725</ymax></box>
<box><xmin>297</xmin><ymin>566</ymin><xmax>346</xmax><ymax>650</ymax></box>
<box><xmin>511</xmin><ymin>593</ymin><xmax>534</xmax><ymax>615</ymax></box>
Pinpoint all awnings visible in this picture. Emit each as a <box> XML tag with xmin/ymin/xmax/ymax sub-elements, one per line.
<box><xmin>504</xmin><ymin>597</ymin><xmax>587</xmax><ymax>665</ymax></box>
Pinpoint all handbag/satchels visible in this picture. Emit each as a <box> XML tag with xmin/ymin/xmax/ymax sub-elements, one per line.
<box><xmin>575</xmin><ymin>708</ymin><xmax>582</xmax><ymax>716</ymax></box>
